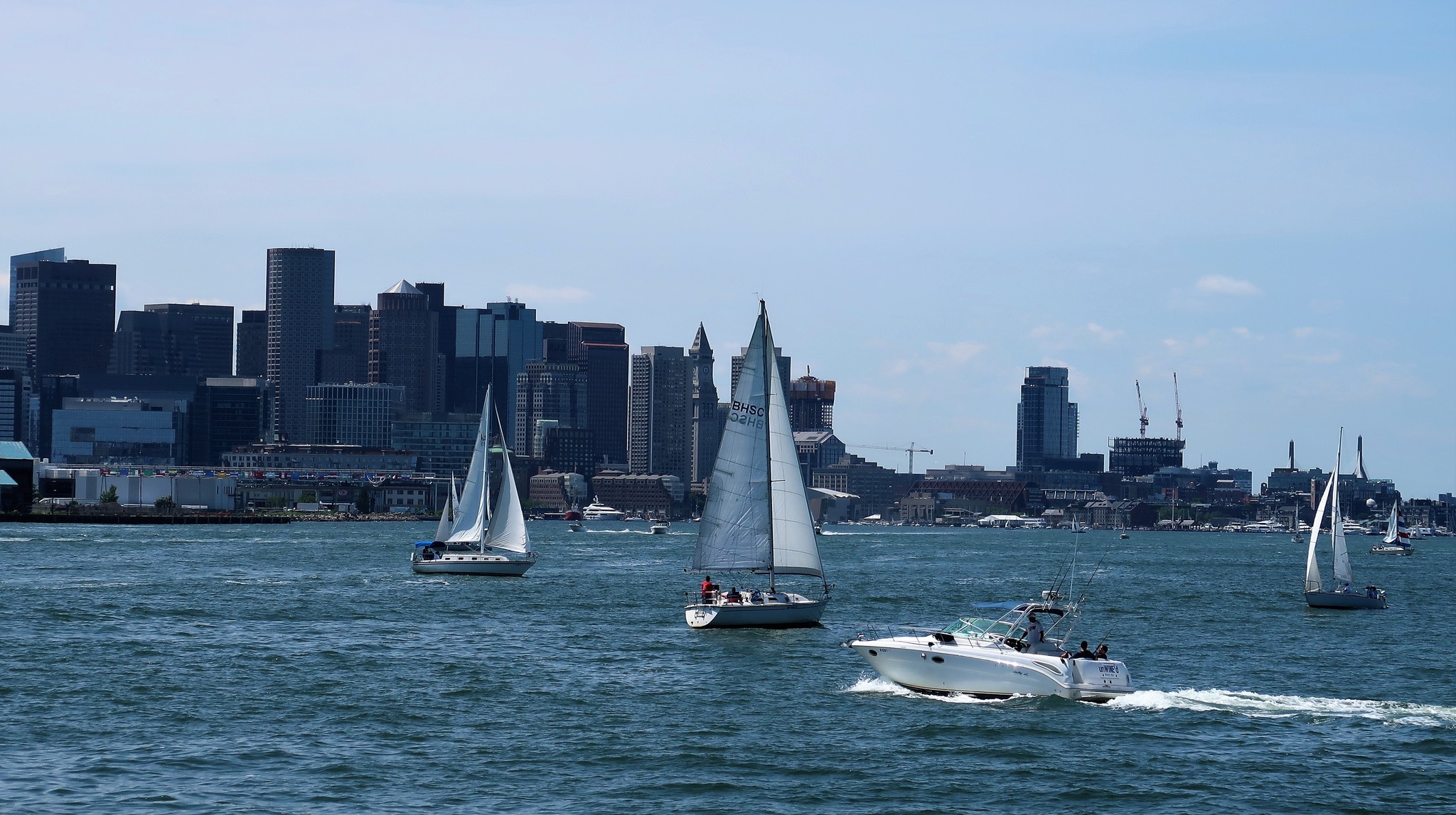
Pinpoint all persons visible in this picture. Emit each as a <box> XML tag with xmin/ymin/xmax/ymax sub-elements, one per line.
<box><xmin>1378</xmin><ymin>545</ymin><xmax>1383</xmax><ymax>550</ymax></box>
<box><xmin>1341</xmin><ymin>583</ymin><xmax>1352</xmax><ymax>592</ymax></box>
<box><xmin>1070</xmin><ymin>640</ymin><xmax>1108</xmax><ymax>660</ymax></box>
<box><xmin>1016</xmin><ymin>613</ymin><xmax>1044</xmax><ymax>647</ymax></box>
<box><xmin>422</xmin><ymin>546</ymin><xmax>440</xmax><ymax>560</ymax></box>
<box><xmin>701</xmin><ymin>575</ymin><xmax>713</xmax><ymax>604</ymax></box>
<box><xmin>1366</xmin><ymin>585</ymin><xmax>1378</xmax><ymax>599</ymax></box>
<box><xmin>578</xmin><ymin>522</ymin><xmax>582</xmax><ymax>526</ymax></box>
<box><xmin>720</xmin><ymin>588</ymin><xmax>743</xmax><ymax>603</ymax></box>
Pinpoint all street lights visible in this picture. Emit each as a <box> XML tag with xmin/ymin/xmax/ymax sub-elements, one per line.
<box><xmin>139</xmin><ymin>474</ymin><xmax>143</xmax><ymax>507</ymax></box>
<box><xmin>36</xmin><ymin>471</ymin><xmax>41</xmax><ymax>504</ymax></box>
<box><xmin>169</xmin><ymin>476</ymin><xmax>174</xmax><ymax>508</ymax></box>
<box><xmin>69</xmin><ymin>473</ymin><xmax>73</xmax><ymax>500</ymax></box>
<box><xmin>196</xmin><ymin>476</ymin><xmax>202</xmax><ymax>509</ymax></box>
<box><xmin>99</xmin><ymin>474</ymin><xmax>105</xmax><ymax>505</ymax></box>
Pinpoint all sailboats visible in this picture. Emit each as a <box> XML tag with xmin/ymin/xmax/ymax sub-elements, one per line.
<box><xmin>1071</xmin><ymin>513</ymin><xmax>1086</xmax><ymax>533</ymax></box>
<box><xmin>1302</xmin><ymin>427</ymin><xmax>1389</xmax><ymax>610</ymax></box>
<box><xmin>1369</xmin><ymin>499</ymin><xmax>1415</xmax><ymax>556</ymax></box>
<box><xmin>1409</xmin><ymin>519</ymin><xmax>1427</xmax><ymax>540</ymax></box>
<box><xmin>684</xmin><ymin>298</ymin><xmax>836</xmax><ymax>629</ymax></box>
<box><xmin>411</xmin><ymin>383</ymin><xmax>538</xmax><ymax>577</ymax></box>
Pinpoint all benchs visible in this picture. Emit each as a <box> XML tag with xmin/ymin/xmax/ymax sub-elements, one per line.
<box><xmin>1020</xmin><ymin>642</ymin><xmax>1109</xmax><ymax>661</ymax></box>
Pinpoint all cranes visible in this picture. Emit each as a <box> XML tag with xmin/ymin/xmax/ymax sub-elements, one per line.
<box><xmin>1174</xmin><ymin>372</ymin><xmax>1183</xmax><ymax>441</ymax></box>
<box><xmin>845</xmin><ymin>442</ymin><xmax>933</xmax><ymax>488</ymax></box>
<box><xmin>1136</xmin><ymin>380</ymin><xmax>1149</xmax><ymax>439</ymax></box>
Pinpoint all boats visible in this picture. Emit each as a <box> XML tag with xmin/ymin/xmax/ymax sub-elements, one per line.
<box><xmin>1120</xmin><ymin>534</ymin><xmax>1130</xmax><ymax>539</ymax></box>
<box><xmin>583</xmin><ymin>495</ymin><xmax>625</xmax><ymax>521</ymax></box>
<box><xmin>838</xmin><ymin>591</ymin><xmax>1136</xmax><ymax>704</ymax></box>
<box><xmin>565</xmin><ymin>502</ymin><xmax>582</xmax><ymax>520</ymax></box>
<box><xmin>650</xmin><ymin>521</ymin><xmax>671</xmax><ymax>533</ymax></box>
<box><xmin>568</xmin><ymin>524</ymin><xmax>587</xmax><ymax>532</ymax></box>
<box><xmin>1224</xmin><ymin>516</ymin><xmax>1453</xmax><ymax>537</ymax></box>
